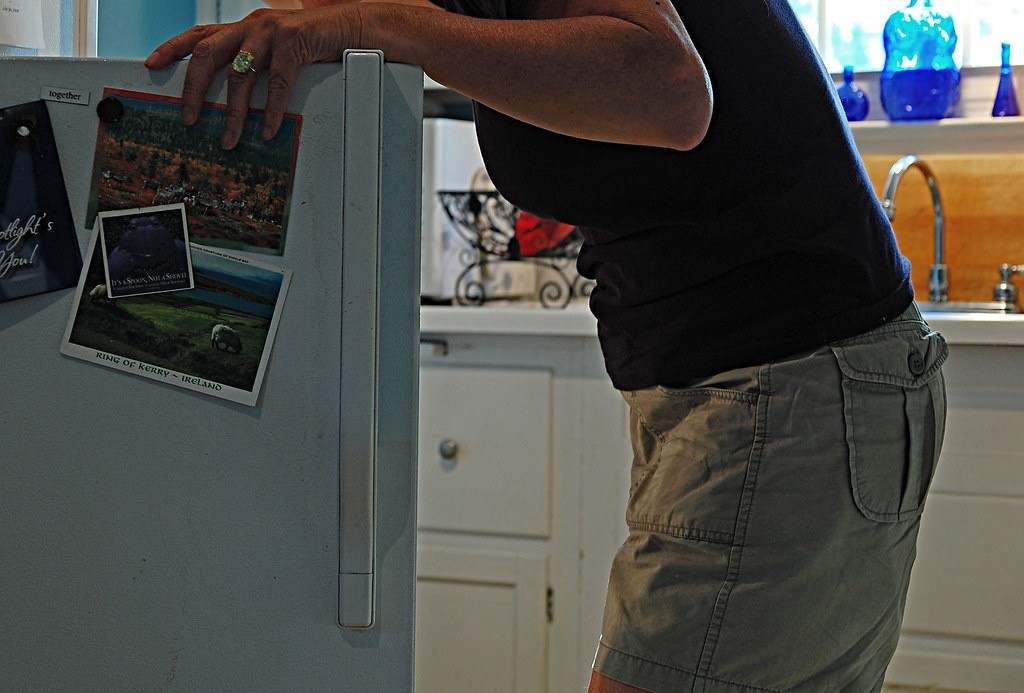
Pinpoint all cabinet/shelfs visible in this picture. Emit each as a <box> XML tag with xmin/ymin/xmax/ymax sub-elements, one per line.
<box><xmin>417</xmin><ymin>330</ymin><xmax>1024</xmax><ymax>693</ymax></box>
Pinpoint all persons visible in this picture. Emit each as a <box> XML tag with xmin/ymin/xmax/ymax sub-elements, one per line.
<box><xmin>146</xmin><ymin>0</ymin><xmax>949</xmax><ymax>693</ymax></box>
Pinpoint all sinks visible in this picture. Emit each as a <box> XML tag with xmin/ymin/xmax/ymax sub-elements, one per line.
<box><xmin>911</xmin><ymin>301</ymin><xmax>1020</xmax><ymax>314</ymax></box>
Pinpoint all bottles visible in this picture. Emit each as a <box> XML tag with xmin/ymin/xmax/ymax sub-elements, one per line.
<box><xmin>836</xmin><ymin>66</ymin><xmax>869</xmax><ymax>121</ymax></box>
<box><xmin>992</xmin><ymin>43</ymin><xmax>1019</xmax><ymax>116</ymax></box>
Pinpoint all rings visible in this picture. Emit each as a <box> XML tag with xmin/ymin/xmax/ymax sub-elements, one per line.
<box><xmin>230</xmin><ymin>49</ymin><xmax>261</xmax><ymax>76</ymax></box>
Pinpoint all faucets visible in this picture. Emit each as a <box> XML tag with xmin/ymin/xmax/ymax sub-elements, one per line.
<box><xmin>880</xmin><ymin>155</ymin><xmax>949</xmax><ymax>302</ymax></box>
